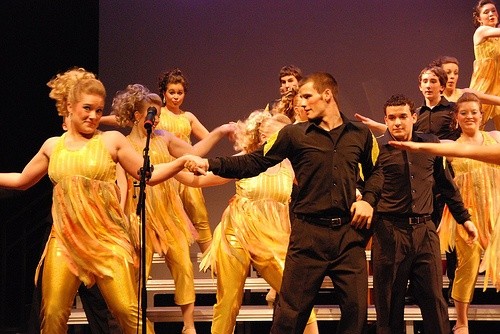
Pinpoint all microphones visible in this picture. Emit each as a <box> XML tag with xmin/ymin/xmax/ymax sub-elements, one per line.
<box><xmin>144</xmin><ymin>106</ymin><xmax>157</xmax><ymax>129</ymax></box>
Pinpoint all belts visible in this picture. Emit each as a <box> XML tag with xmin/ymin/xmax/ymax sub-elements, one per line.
<box><xmin>293</xmin><ymin>213</ymin><xmax>349</xmax><ymax>228</ymax></box>
<box><xmin>379</xmin><ymin>214</ymin><xmax>432</xmax><ymax>225</ymax></box>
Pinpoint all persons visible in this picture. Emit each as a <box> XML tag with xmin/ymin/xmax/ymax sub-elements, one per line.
<box><xmin>111</xmin><ymin>83</ymin><xmax>236</xmax><ymax>334</ymax></box>
<box><xmin>469</xmin><ymin>0</ymin><xmax>500</xmax><ymax>131</ymax></box>
<box><xmin>433</xmin><ymin>56</ymin><xmax>500</xmax><ymax>105</ymax></box>
<box><xmin>183</xmin><ymin>72</ymin><xmax>385</xmax><ymax>334</ymax></box>
<box><xmin>405</xmin><ymin>63</ymin><xmax>455</xmax><ymax>306</ymax></box>
<box><xmin>389</xmin><ymin>141</ymin><xmax>500</xmax><ymax>164</ymax></box>
<box><xmin>356</xmin><ymin>95</ymin><xmax>477</xmax><ymax>334</ymax></box>
<box><xmin>354</xmin><ymin>93</ymin><xmax>500</xmax><ymax>334</ymax></box>
<box><xmin>0</xmin><ymin>68</ymin><xmax>207</xmax><ymax>334</ymax></box>
<box><xmin>267</xmin><ymin>64</ymin><xmax>309</xmax><ymax>125</ymax></box>
<box><xmin>173</xmin><ymin>111</ymin><xmax>319</xmax><ymax>334</ymax></box>
<box><xmin>98</xmin><ymin>69</ymin><xmax>217</xmax><ymax>278</ymax></box>
